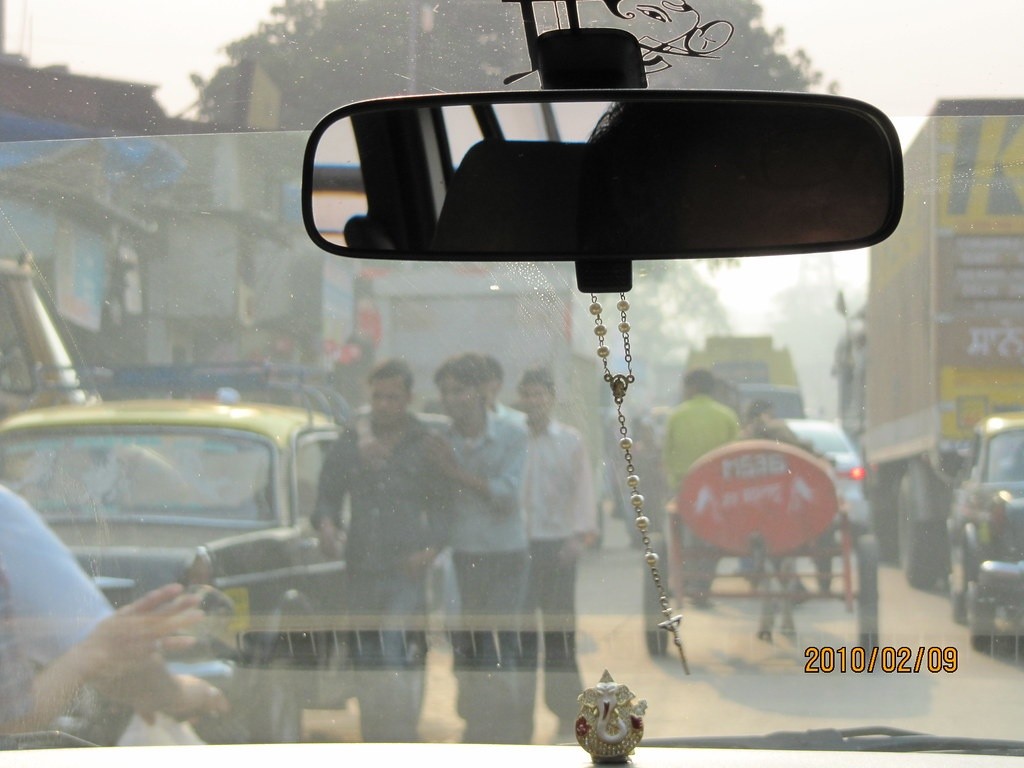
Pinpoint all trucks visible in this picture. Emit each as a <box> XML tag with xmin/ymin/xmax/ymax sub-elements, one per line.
<box><xmin>832</xmin><ymin>101</ymin><xmax>1023</xmax><ymax>588</ymax></box>
<box><xmin>678</xmin><ymin>328</ymin><xmax>798</xmax><ymax>436</ymax></box>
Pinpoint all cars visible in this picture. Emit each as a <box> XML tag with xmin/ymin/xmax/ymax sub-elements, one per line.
<box><xmin>943</xmin><ymin>410</ymin><xmax>1022</xmax><ymax>647</ymax></box>
<box><xmin>1</xmin><ymin>398</ymin><xmax>355</xmax><ymax>740</ymax></box>
<box><xmin>782</xmin><ymin>421</ymin><xmax>862</xmax><ymax>509</ymax></box>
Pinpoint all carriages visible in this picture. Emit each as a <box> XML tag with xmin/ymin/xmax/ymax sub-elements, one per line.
<box><xmin>637</xmin><ymin>406</ymin><xmax>876</xmax><ymax>669</ymax></box>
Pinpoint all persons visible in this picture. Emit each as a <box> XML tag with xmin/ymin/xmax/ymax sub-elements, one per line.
<box><xmin>0</xmin><ymin>567</ymin><xmax>232</xmax><ymax>750</ymax></box>
<box><xmin>351</xmin><ymin>350</ymin><xmax>536</xmax><ymax>745</ymax></box>
<box><xmin>512</xmin><ymin>366</ymin><xmax>598</xmax><ymax>739</ymax></box>
<box><xmin>551</xmin><ymin>377</ymin><xmax>754</xmax><ymax>556</ymax></box>
<box><xmin>1</xmin><ymin>484</ymin><xmax>235</xmax><ymax>750</ymax></box>
<box><xmin>433</xmin><ymin>356</ymin><xmax>530</xmax><ymax>721</ymax></box>
<box><xmin>309</xmin><ymin>359</ymin><xmax>464</xmax><ymax>745</ymax></box>
<box><xmin>661</xmin><ymin>365</ymin><xmax>741</xmax><ymax>611</ymax></box>
<box><xmin>577</xmin><ymin>93</ymin><xmax>896</xmax><ymax>260</ymax></box>
